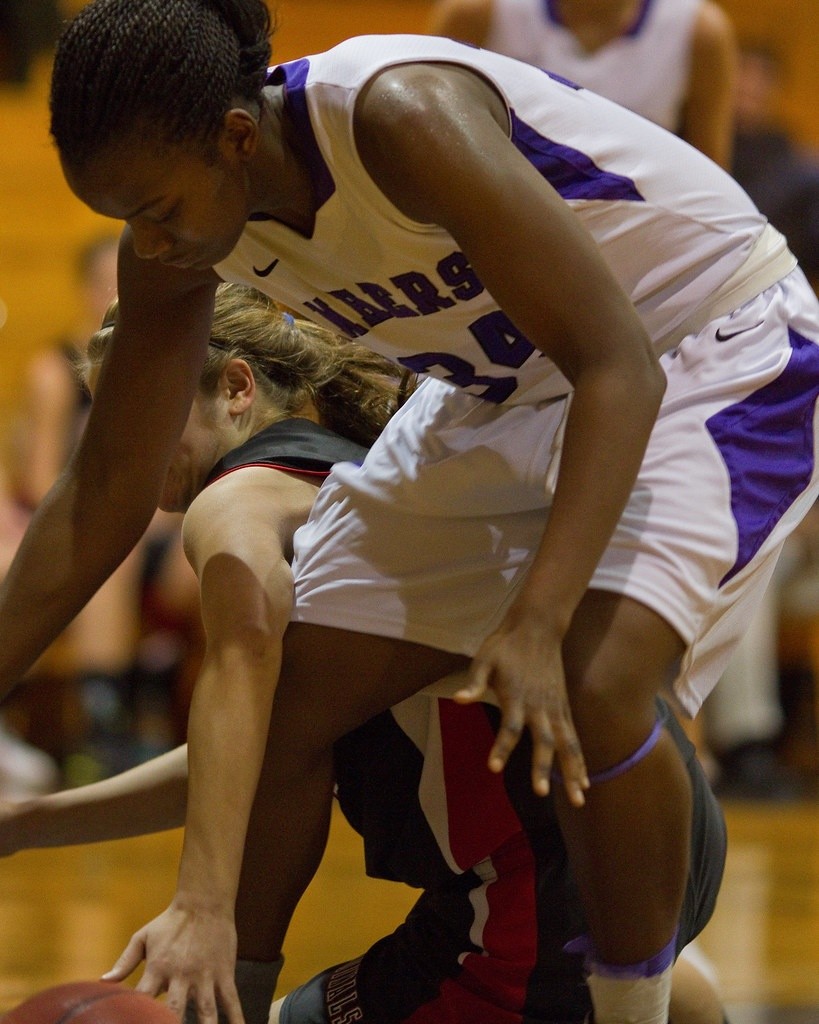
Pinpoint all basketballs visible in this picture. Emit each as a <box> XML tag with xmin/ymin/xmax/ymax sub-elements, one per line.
<box><xmin>0</xmin><ymin>977</ymin><xmax>184</xmax><ymax>1024</ymax></box>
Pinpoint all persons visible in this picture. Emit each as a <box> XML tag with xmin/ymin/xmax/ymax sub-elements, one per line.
<box><xmin>0</xmin><ymin>0</ymin><xmax>819</xmax><ymax>1024</ymax></box>
